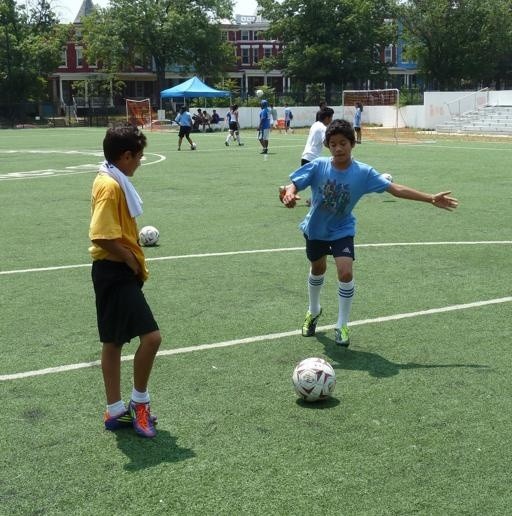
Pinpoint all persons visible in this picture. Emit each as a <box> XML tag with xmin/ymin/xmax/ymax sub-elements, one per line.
<box><xmin>279</xmin><ymin>107</ymin><xmax>334</xmax><ymax>207</ymax></box>
<box><xmin>281</xmin><ymin>120</ymin><xmax>458</xmax><ymax>347</ymax></box>
<box><xmin>87</xmin><ymin>121</ymin><xmax>161</xmax><ymax>440</ymax></box>
<box><xmin>172</xmin><ymin>99</ymin><xmax>364</xmax><ymax>155</ymax></box>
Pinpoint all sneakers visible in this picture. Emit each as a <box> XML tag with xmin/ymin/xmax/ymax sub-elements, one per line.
<box><xmin>305</xmin><ymin>198</ymin><xmax>311</xmax><ymax>206</ymax></box>
<box><xmin>335</xmin><ymin>325</ymin><xmax>350</xmax><ymax>346</ymax></box>
<box><xmin>302</xmin><ymin>308</ymin><xmax>322</xmax><ymax>337</ymax></box>
<box><xmin>104</xmin><ymin>410</ymin><xmax>157</xmax><ymax>430</ymax></box>
<box><xmin>279</xmin><ymin>186</ymin><xmax>287</xmax><ymax>203</ymax></box>
<box><xmin>128</xmin><ymin>400</ymin><xmax>156</xmax><ymax>437</ymax></box>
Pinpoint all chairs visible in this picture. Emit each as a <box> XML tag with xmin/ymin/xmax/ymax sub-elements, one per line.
<box><xmin>275</xmin><ymin>119</ymin><xmax>289</xmax><ymax>133</ymax></box>
<box><xmin>193</xmin><ymin>116</ymin><xmax>226</xmax><ymax>133</ymax></box>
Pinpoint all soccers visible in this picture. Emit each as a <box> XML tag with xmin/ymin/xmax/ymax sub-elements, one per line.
<box><xmin>139</xmin><ymin>226</ymin><xmax>160</xmax><ymax>246</ymax></box>
<box><xmin>379</xmin><ymin>173</ymin><xmax>393</xmax><ymax>183</ymax></box>
<box><xmin>191</xmin><ymin>143</ymin><xmax>197</xmax><ymax>149</ymax></box>
<box><xmin>257</xmin><ymin>90</ymin><xmax>263</xmax><ymax>97</ymax></box>
<box><xmin>293</xmin><ymin>358</ymin><xmax>335</xmax><ymax>401</ymax></box>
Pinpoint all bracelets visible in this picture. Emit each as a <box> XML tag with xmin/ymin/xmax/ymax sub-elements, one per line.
<box><xmin>431</xmin><ymin>195</ymin><xmax>436</xmax><ymax>205</ymax></box>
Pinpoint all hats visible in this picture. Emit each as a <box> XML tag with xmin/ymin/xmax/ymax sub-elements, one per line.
<box><xmin>261</xmin><ymin>100</ymin><xmax>268</xmax><ymax>106</ymax></box>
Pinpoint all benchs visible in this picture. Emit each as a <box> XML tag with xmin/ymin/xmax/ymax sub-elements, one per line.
<box><xmin>435</xmin><ymin>101</ymin><xmax>512</xmax><ymax>134</ymax></box>
